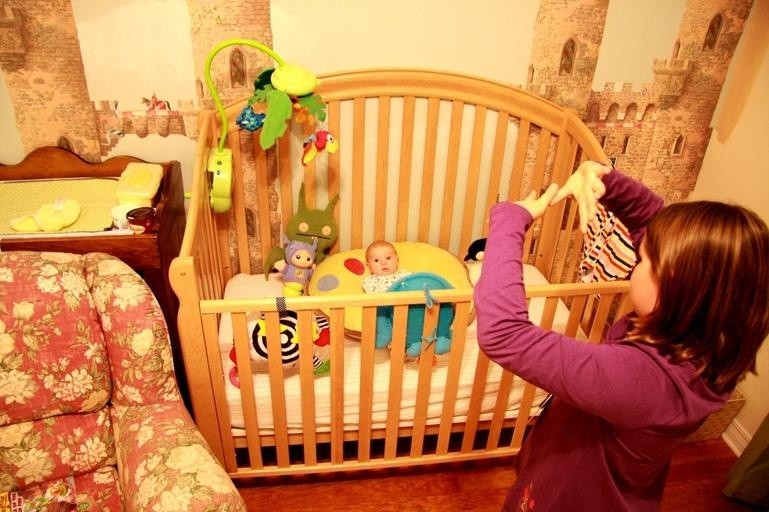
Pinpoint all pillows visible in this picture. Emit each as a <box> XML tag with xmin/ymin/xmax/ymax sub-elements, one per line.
<box><xmin>304</xmin><ymin>242</ymin><xmax>479</xmax><ymax>336</ymax></box>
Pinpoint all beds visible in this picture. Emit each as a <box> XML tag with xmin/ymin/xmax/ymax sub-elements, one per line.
<box><xmin>170</xmin><ymin>69</ymin><xmax>651</xmax><ymax>480</ymax></box>
<box><xmin>0</xmin><ymin>144</ymin><xmax>184</xmax><ymax>323</ymax></box>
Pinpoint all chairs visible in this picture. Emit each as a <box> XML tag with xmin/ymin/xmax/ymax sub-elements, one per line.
<box><xmin>0</xmin><ymin>246</ymin><xmax>247</xmax><ymax>512</ymax></box>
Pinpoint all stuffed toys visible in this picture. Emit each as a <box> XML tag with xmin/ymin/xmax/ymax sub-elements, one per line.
<box><xmin>374</xmin><ymin>271</ymin><xmax>458</xmax><ymax>362</ymax></box>
<box><xmin>463</xmin><ymin>238</ymin><xmax>488</xmax><ymax>264</ymax></box>
<box><xmin>228</xmin><ymin>296</ymin><xmax>335</xmax><ymax>389</ymax></box>
<box><xmin>264</xmin><ymin>182</ymin><xmax>341</xmax><ymax>282</ymax></box>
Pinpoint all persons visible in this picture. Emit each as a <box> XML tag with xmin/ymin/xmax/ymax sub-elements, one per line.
<box><xmin>362</xmin><ymin>240</ymin><xmax>438</xmax><ymax>369</ymax></box>
<box><xmin>472</xmin><ymin>161</ymin><xmax>768</xmax><ymax>511</ymax></box>
<box><xmin>273</xmin><ymin>239</ymin><xmax>322</xmax><ymax>298</ymax></box>
